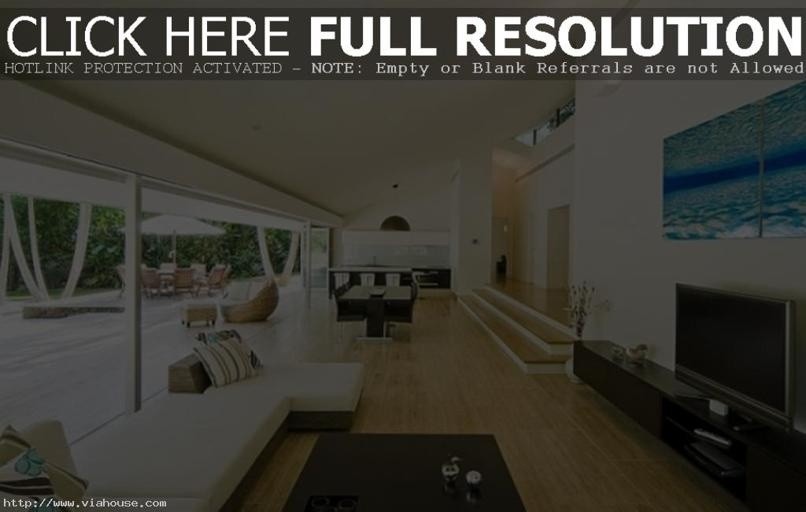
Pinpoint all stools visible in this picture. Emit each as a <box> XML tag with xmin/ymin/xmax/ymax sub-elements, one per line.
<box><xmin>176</xmin><ymin>304</ymin><xmax>217</xmax><ymax>328</ymax></box>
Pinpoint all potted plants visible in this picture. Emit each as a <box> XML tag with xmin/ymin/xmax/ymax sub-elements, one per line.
<box><xmin>560</xmin><ymin>281</ymin><xmax>612</xmax><ymax>383</ymax></box>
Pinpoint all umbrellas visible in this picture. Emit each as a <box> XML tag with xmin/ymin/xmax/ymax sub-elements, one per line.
<box><xmin>118</xmin><ymin>215</ymin><xmax>226</xmax><ymax>262</ymax></box>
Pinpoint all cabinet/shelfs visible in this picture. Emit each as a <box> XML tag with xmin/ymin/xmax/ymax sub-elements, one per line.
<box><xmin>329</xmin><ymin>271</ymin><xmax>412</xmax><ymax>299</ymax></box>
<box><xmin>573</xmin><ymin>340</ymin><xmax>806</xmax><ymax>511</ymax></box>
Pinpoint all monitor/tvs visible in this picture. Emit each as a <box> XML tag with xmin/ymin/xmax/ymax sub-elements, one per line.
<box><xmin>675</xmin><ymin>283</ymin><xmax>796</xmax><ymax>432</ymax></box>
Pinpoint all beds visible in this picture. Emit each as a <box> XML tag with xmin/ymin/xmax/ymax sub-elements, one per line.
<box><xmin>0</xmin><ymin>394</ymin><xmax>290</xmax><ymax>512</ymax></box>
<box><xmin>281</xmin><ymin>432</ymin><xmax>527</xmax><ymax>512</ymax></box>
<box><xmin>168</xmin><ymin>354</ymin><xmax>364</xmax><ymax>430</ymax></box>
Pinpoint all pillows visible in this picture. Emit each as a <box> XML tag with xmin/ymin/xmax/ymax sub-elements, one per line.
<box><xmin>0</xmin><ymin>424</ymin><xmax>89</xmax><ymax>512</ymax></box>
<box><xmin>229</xmin><ymin>281</ymin><xmax>249</xmax><ymax>300</ymax></box>
<box><xmin>248</xmin><ymin>280</ymin><xmax>268</xmax><ymax>300</ymax></box>
<box><xmin>194</xmin><ymin>338</ymin><xmax>255</xmax><ymax>388</ymax></box>
<box><xmin>194</xmin><ymin>329</ymin><xmax>262</xmax><ymax>369</ymax></box>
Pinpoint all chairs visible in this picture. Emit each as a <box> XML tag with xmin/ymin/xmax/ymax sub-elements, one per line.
<box><xmin>219</xmin><ymin>276</ymin><xmax>279</xmax><ymax>321</ymax></box>
<box><xmin>334</xmin><ymin>282</ymin><xmax>417</xmax><ymax>342</ymax></box>
<box><xmin>115</xmin><ymin>262</ymin><xmax>226</xmax><ymax>300</ymax></box>
